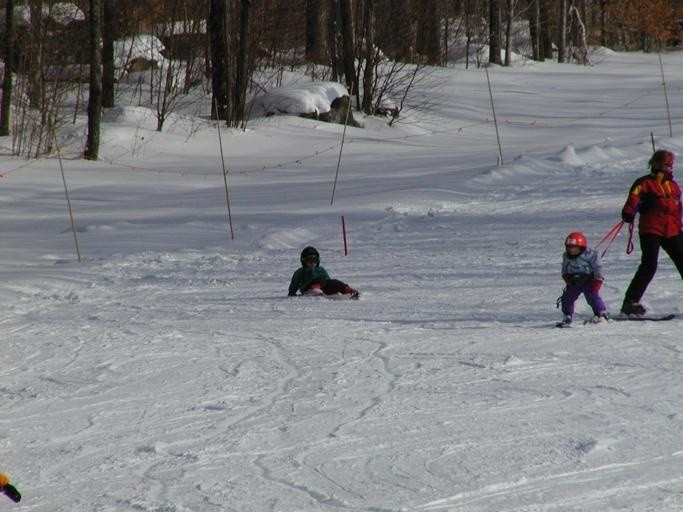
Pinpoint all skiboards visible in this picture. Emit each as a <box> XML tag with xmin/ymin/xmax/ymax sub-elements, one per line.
<box><xmin>571</xmin><ymin>311</ymin><xmax>683</xmax><ymax>321</ymax></box>
<box><xmin>302</xmin><ymin>291</ymin><xmax>359</xmax><ymax>299</ymax></box>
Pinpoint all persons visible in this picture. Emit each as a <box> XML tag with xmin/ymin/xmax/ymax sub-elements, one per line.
<box><xmin>285</xmin><ymin>246</ymin><xmax>358</xmax><ymax>300</ymax></box>
<box><xmin>552</xmin><ymin>231</ymin><xmax>611</xmax><ymax>329</ymax></box>
<box><xmin>616</xmin><ymin>148</ymin><xmax>683</xmax><ymax>315</ymax></box>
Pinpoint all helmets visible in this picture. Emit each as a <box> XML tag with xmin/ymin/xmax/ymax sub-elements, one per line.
<box><xmin>564</xmin><ymin>232</ymin><xmax>588</xmax><ymax>248</ymax></box>
<box><xmin>300</xmin><ymin>246</ymin><xmax>320</xmax><ymax>268</ymax></box>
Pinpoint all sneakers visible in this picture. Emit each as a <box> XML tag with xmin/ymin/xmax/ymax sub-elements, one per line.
<box><xmin>555</xmin><ymin>316</ymin><xmax>572</xmax><ymax>328</ymax></box>
<box><xmin>602</xmin><ymin>312</ymin><xmax>610</xmax><ymax>320</ymax></box>
<box><xmin>306</xmin><ymin>283</ymin><xmax>324</xmax><ymax>295</ymax></box>
<box><xmin>337</xmin><ymin>284</ymin><xmax>359</xmax><ymax>297</ymax></box>
<box><xmin>620</xmin><ymin>302</ymin><xmax>646</xmax><ymax>317</ymax></box>
